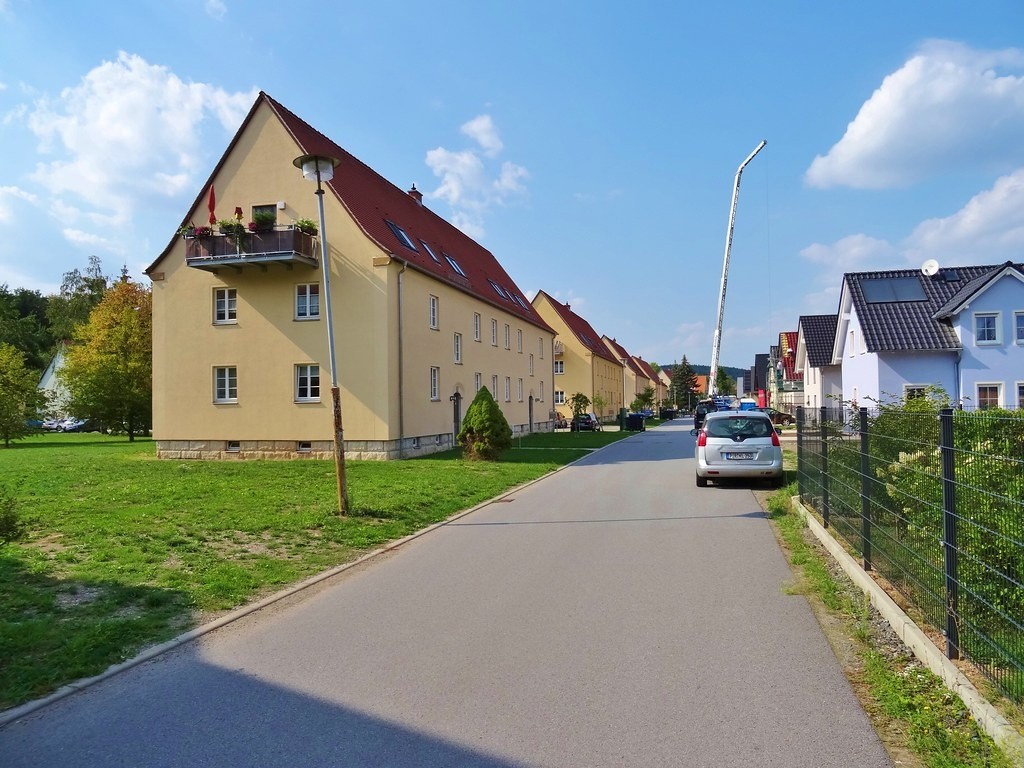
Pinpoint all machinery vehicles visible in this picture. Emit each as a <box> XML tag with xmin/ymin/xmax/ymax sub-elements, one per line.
<box><xmin>707</xmin><ymin>140</ymin><xmax>771</xmax><ymax>411</ymax></box>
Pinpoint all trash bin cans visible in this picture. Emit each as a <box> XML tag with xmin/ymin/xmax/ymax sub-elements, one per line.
<box><xmin>628</xmin><ymin>413</ymin><xmax>646</xmax><ymax>432</ymax></box>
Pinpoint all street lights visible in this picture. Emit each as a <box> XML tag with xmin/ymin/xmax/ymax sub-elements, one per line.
<box><xmin>292</xmin><ymin>154</ymin><xmax>349</xmax><ymax>516</ymax></box>
<box><xmin>659</xmin><ymin>379</ymin><xmax>663</xmax><ymax>407</ymax></box>
<box><xmin>688</xmin><ymin>392</ymin><xmax>690</xmax><ymax>410</ymax></box>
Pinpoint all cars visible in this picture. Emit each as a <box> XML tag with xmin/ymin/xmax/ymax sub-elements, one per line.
<box><xmin>42</xmin><ymin>419</ymin><xmax>66</xmax><ymax>431</ymax></box>
<box><xmin>746</xmin><ymin>407</ymin><xmax>796</xmax><ymax>426</ymax></box>
<box><xmin>62</xmin><ymin>417</ymin><xmax>100</xmax><ymax>433</ymax></box>
<box><xmin>26</xmin><ymin>418</ymin><xmax>51</xmax><ymax>429</ymax></box>
<box><xmin>694</xmin><ymin>405</ymin><xmax>718</xmax><ymax>429</ymax></box>
<box><xmin>690</xmin><ymin>412</ymin><xmax>782</xmax><ymax>487</ymax></box>
<box><xmin>644</xmin><ymin>410</ymin><xmax>654</xmax><ymax>419</ymax></box>
<box><xmin>571</xmin><ymin>413</ymin><xmax>601</xmax><ymax>432</ymax></box>
<box><xmin>555</xmin><ymin>412</ymin><xmax>567</xmax><ymax>429</ymax></box>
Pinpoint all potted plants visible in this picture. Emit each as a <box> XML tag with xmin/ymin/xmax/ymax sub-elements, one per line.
<box><xmin>218</xmin><ymin>220</ymin><xmax>236</xmax><ymax>233</ymax></box>
<box><xmin>253</xmin><ymin>209</ymin><xmax>277</xmax><ymax>231</ymax></box>
<box><xmin>294</xmin><ymin>218</ymin><xmax>318</xmax><ymax>235</ymax></box>
